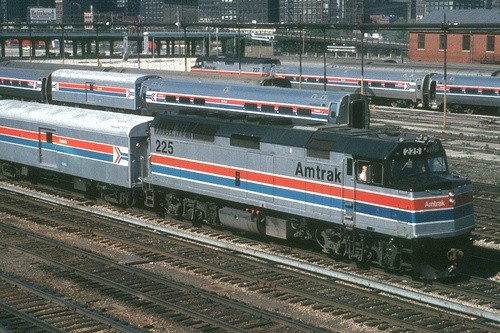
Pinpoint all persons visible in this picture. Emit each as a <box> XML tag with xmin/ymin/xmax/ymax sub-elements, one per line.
<box><xmin>360</xmin><ymin>163</ymin><xmax>374</xmax><ymax>183</ymax></box>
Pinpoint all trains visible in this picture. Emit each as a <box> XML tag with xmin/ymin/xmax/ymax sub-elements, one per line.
<box><xmin>190</xmin><ymin>53</ymin><xmax>500</xmax><ymax>116</ymax></box>
<box><xmin>0</xmin><ymin>99</ymin><xmax>475</xmax><ymax>280</ymax></box>
<box><xmin>0</xmin><ymin>59</ymin><xmax>382</xmax><ymax>126</ymax></box>
